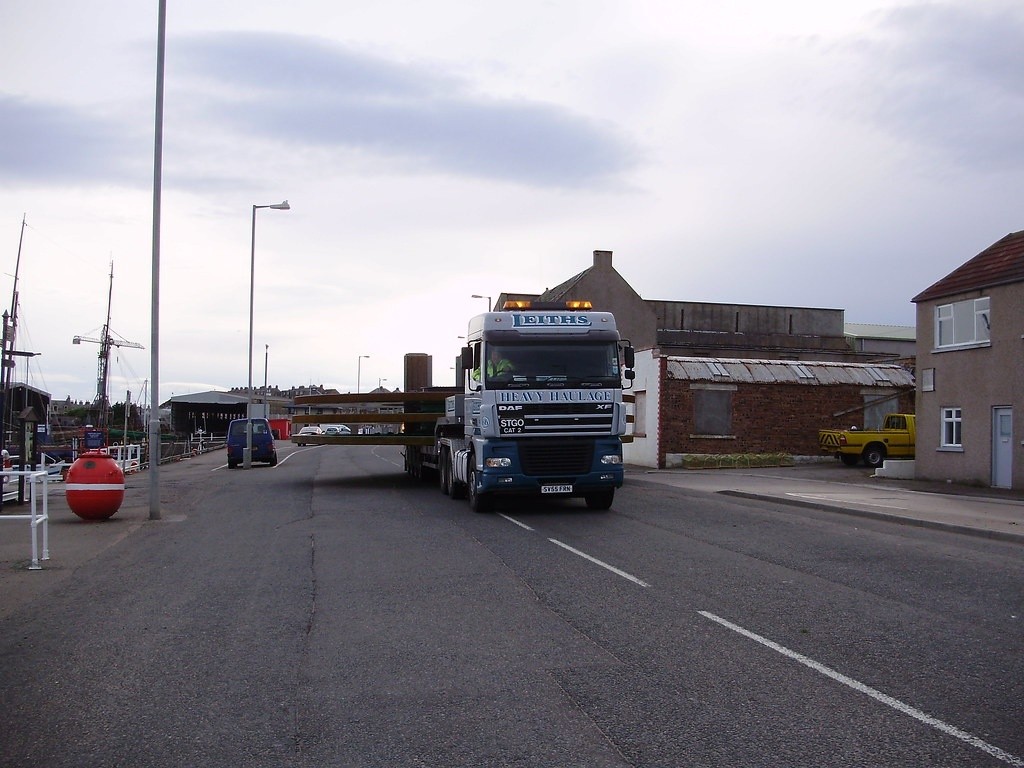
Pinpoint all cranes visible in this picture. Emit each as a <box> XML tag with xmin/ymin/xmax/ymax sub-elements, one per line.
<box><xmin>72</xmin><ymin>251</ymin><xmax>145</xmax><ymax>448</ymax></box>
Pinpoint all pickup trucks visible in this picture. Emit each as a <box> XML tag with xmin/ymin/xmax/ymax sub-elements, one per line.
<box><xmin>818</xmin><ymin>414</ymin><xmax>916</xmax><ymax>468</ymax></box>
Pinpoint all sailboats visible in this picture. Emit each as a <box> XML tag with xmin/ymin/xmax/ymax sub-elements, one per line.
<box><xmin>0</xmin><ymin>212</ymin><xmax>68</xmax><ymax>484</ymax></box>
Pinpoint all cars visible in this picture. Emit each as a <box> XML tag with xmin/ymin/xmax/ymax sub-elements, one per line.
<box><xmin>326</xmin><ymin>427</ymin><xmax>341</xmax><ymax>435</ymax></box>
<box><xmin>297</xmin><ymin>427</ymin><xmax>326</xmax><ymax>446</ymax></box>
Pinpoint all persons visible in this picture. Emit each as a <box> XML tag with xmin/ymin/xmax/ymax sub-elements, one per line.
<box><xmin>472</xmin><ymin>348</ymin><xmax>518</xmax><ymax>382</ymax></box>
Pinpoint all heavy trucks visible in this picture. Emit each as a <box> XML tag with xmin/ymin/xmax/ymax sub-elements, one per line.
<box><xmin>400</xmin><ymin>300</ymin><xmax>636</xmax><ymax>513</ymax></box>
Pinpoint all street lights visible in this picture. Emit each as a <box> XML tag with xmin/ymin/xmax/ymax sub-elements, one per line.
<box><xmin>377</xmin><ymin>378</ymin><xmax>388</xmax><ymax>435</ymax></box>
<box><xmin>356</xmin><ymin>355</ymin><xmax>370</xmax><ymax>415</ymax></box>
<box><xmin>471</xmin><ymin>295</ymin><xmax>491</xmax><ymax>312</ymax></box>
<box><xmin>242</xmin><ymin>199</ymin><xmax>290</xmax><ymax>469</ymax></box>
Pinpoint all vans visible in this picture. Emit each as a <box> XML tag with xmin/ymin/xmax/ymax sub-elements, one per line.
<box><xmin>328</xmin><ymin>425</ymin><xmax>351</xmax><ymax>435</ymax></box>
<box><xmin>226</xmin><ymin>417</ymin><xmax>278</xmax><ymax>469</ymax></box>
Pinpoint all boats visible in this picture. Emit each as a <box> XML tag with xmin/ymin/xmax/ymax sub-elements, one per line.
<box><xmin>100</xmin><ymin>441</ymin><xmax>141</xmax><ymax>474</ymax></box>
